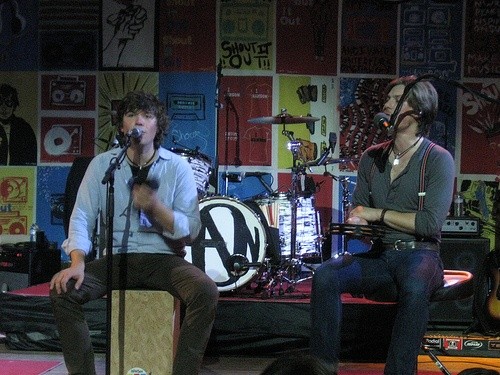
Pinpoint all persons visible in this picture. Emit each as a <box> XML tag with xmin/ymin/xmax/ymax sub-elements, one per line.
<box><xmin>311</xmin><ymin>76</ymin><xmax>455</xmax><ymax>375</ymax></box>
<box><xmin>0</xmin><ymin>83</ymin><xmax>36</xmax><ymax>166</ymax></box>
<box><xmin>260</xmin><ymin>355</ymin><xmax>337</xmax><ymax>375</ymax></box>
<box><xmin>50</xmin><ymin>90</ymin><xmax>219</xmax><ymax>375</ymax></box>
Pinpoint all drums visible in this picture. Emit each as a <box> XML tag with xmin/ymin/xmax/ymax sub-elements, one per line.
<box><xmin>171</xmin><ymin>149</ymin><xmax>213</xmax><ymax>199</ymax></box>
<box><xmin>180</xmin><ymin>198</ymin><xmax>269</xmax><ymax>291</ymax></box>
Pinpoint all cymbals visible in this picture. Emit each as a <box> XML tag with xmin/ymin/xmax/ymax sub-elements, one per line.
<box><xmin>288</xmin><ymin>158</ymin><xmax>351</xmax><ymax>169</ymax></box>
<box><xmin>328</xmin><ymin>222</ymin><xmax>388</xmax><ymax>237</ymax></box>
<box><xmin>245</xmin><ymin>116</ymin><xmax>322</xmax><ymax>123</ymax></box>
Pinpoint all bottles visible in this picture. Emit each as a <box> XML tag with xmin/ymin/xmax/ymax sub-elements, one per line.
<box><xmin>31</xmin><ymin>224</ymin><xmax>39</xmax><ymax>242</ymax></box>
<box><xmin>453</xmin><ymin>192</ymin><xmax>463</xmax><ymax>217</ymax></box>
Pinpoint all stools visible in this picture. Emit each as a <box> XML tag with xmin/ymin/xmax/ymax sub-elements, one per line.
<box><xmin>110</xmin><ymin>289</ymin><xmax>181</xmax><ymax>374</ymax></box>
<box><xmin>350</xmin><ymin>269</ymin><xmax>475</xmax><ymax>375</ymax></box>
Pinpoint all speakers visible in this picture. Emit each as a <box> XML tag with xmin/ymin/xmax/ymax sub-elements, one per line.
<box><xmin>425</xmin><ymin>234</ymin><xmax>490</xmax><ymax>331</ymax></box>
<box><xmin>0</xmin><ymin>244</ymin><xmax>61</xmax><ymax>293</ymax></box>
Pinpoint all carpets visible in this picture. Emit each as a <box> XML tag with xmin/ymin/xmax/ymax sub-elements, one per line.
<box><xmin>0</xmin><ymin>359</ymin><xmax>64</xmax><ymax>375</ymax></box>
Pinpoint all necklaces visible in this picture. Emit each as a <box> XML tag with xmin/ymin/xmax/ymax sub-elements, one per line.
<box><xmin>392</xmin><ymin>135</ymin><xmax>423</xmax><ymax>165</ymax></box>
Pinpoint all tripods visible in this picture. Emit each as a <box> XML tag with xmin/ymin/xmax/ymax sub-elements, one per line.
<box><xmin>259</xmin><ymin>123</ymin><xmax>316</xmax><ymax>299</ymax></box>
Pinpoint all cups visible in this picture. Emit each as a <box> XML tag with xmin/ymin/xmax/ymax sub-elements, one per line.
<box><xmin>36</xmin><ymin>230</ymin><xmax>46</xmax><ymax>243</ymax></box>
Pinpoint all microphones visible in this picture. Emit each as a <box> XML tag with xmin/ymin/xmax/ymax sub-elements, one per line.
<box><xmin>231</xmin><ymin>260</ymin><xmax>241</xmax><ymax>273</ymax></box>
<box><xmin>241</xmin><ymin>171</ymin><xmax>268</xmax><ymax>178</ymax></box>
<box><xmin>329</xmin><ymin>132</ymin><xmax>337</xmax><ymax>154</ymax></box>
<box><xmin>214</xmin><ymin>60</ymin><xmax>223</xmax><ymax>107</ymax></box>
<box><xmin>374</xmin><ymin>113</ymin><xmax>395</xmax><ymax>137</ymax></box>
<box><xmin>127</xmin><ymin>128</ymin><xmax>142</xmax><ymax>138</ymax></box>
